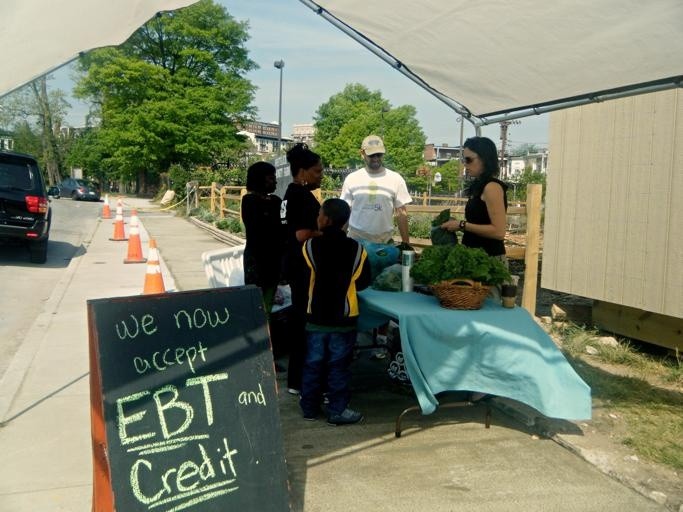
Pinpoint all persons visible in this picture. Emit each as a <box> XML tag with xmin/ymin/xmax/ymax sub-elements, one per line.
<box><xmin>277</xmin><ymin>142</ymin><xmax>327</xmax><ymax>398</ymax></box>
<box><xmin>334</xmin><ymin>133</ymin><xmax>413</xmax><ymax>249</ymax></box>
<box><xmin>237</xmin><ymin>161</ymin><xmax>287</xmax><ymax>317</ymax></box>
<box><xmin>296</xmin><ymin>197</ymin><xmax>370</xmax><ymax>429</ymax></box>
<box><xmin>439</xmin><ymin>134</ymin><xmax>509</xmax><ymax>282</ymax></box>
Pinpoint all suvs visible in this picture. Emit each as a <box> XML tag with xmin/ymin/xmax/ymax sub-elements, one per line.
<box><xmin>0</xmin><ymin>148</ymin><xmax>60</xmax><ymax>264</ymax></box>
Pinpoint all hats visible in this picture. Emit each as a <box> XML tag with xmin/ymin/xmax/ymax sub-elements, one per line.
<box><xmin>361</xmin><ymin>135</ymin><xmax>386</xmax><ymax>156</ymax></box>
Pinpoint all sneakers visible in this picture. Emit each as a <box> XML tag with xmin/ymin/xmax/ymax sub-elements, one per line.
<box><xmin>327</xmin><ymin>407</ymin><xmax>365</xmax><ymax>427</ymax></box>
<box><xmin>288</xmin><ymin>388</ymin><xmax>301</xmax><ymax>394</ymax></box>
<box><xmin>300</xmin><ymin>392</ymin><xmax>330</xmax><ymax>405</ymax></box>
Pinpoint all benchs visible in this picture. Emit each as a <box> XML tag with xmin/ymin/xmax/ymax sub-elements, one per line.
<box><xmin>196</xmin><ymin>245</ymin><xmax>294</xmax><ymax>374</ymax></box>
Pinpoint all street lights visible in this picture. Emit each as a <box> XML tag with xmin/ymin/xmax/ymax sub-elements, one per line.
<box><xmin>274</xmin><ymin>59</ymin><xmax>284</xmax><ymax>156</ymax></box>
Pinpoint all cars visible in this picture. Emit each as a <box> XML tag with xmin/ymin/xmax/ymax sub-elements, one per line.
<box><xmin>53</xmin><ymin>175</ymin><xmax>102</xmax><ymax>201</ymax></box>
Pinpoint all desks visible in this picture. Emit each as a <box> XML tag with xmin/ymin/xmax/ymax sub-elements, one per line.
<box><xmin>356</xmin><ymin>292</ymin><xmax>593</xmax><ymax>438</ymax></box>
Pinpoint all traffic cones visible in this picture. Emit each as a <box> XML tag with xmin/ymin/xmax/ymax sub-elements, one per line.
<box><xmin>126</xmin><ymin>208</ymin><xmax>148</xmax><ymax>262</ymax></box>
<box><xmin>110</xmin><ymin>203</ymin><xmax>128</xmax><ymax>242</ymax></box>
<box><xmin>100</xmin><ymin>193</ymin><xmax>113</xmax><ymax>221</ymax></box>
<box><xmin>143</xmin><ymin>236</ymin><xmax>167</xmax><ymax>295</ymax></box>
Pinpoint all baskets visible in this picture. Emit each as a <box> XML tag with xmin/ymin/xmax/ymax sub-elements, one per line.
<box><xmin>428</xmin><ymin>278</ymin><xmax>495</xmax><ymax>310</ymax></box>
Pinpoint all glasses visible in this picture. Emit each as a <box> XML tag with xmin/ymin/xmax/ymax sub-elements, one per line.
<box><xmin>461</xmin><ymin>156</ymin><xmax>474</xmax><ymax>163</ymax></box>
<box><xmin>368</xmin><ymin>153</ymin><xmax>383</xmax><ymax>158</ymax></box>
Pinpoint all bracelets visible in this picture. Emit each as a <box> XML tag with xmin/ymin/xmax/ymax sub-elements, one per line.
<box><xmin>459</xmin><ymin>220</ymin><xmax>466</xmax><ymax>233</ymax></box>
